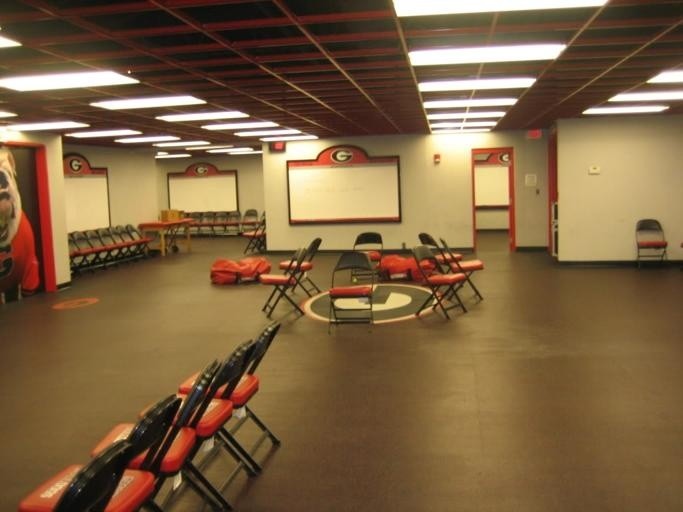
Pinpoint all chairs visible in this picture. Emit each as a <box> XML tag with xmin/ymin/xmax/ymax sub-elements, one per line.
<box><xmin>261</xmin><ymin>237</ymin><xmax>322</xmax><ymax>319</ymax></box>
<box><xmin>68</xmin><ymin>224</ymin><xmax>153</xmax><ymax>278</ymax></box>
<box><xmin>18</xmin><ymin>319</ymin><xmax>282</xmax><ymax>511</ymax></box>
<box><xmin>328</xmin><ymin>233</ymin><xmax>485</xmax><ymax>335</ymax></box>
<box><xmin>181</xmin><ymin>209</ymin><xmax>265</xmax><ymax>240</ymax></box>
<box><xmin>243</xmin><ymin>219</ymin><xmax>266</xmax><ymax>255</ymax></box>
<box><xmin>635</xmin><ymin>219</ymin><xmax>669</xmax><ymax>271</ymax></box>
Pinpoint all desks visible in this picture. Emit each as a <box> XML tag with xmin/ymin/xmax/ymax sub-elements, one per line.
<box><xmin>139</xmin><ymin>218</ymin><xmax>191</xmax><ymax>256</ymax></box>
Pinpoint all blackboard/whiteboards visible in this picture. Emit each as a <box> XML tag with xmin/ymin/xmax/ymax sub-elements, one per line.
<box><xmin>167</xmin><ymin>162</ymin><xmax>239</xmax><ymax>214</ymax></box>
<box><xmin>473</xmin><ymin>153</ymin><xmax>509</xmax><ymax>208</ymax></box>
<box><xmin>286</xmin><ymin>144</ymin><xmax>400</xmax><ymax>224</ymax></box>
<box><xmin>63</xmin><ymin>153</ymin><xmax>110</xmax><ymax>234</ymax></box>
